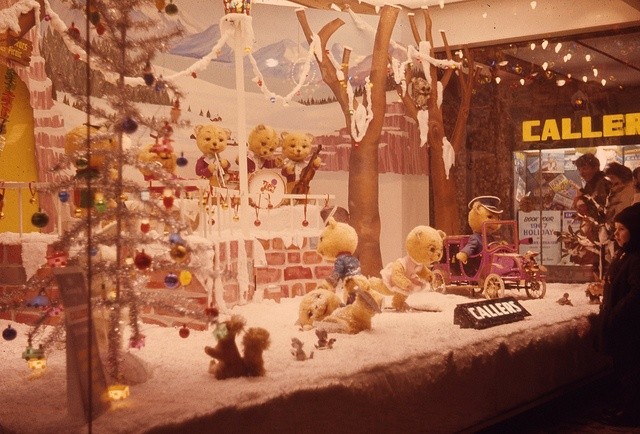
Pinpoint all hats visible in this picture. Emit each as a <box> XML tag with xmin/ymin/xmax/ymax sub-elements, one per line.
<box><xmin>613</xmin><ymin>201</ymin><xmax>640</xmax><ymax>238</ymax></box>
<box><xmin>467</xmin><ymin>196</ymin><xmax>503</xmax><ymax>214</ymax></box>
<box><xmin>342</xmin><ymin>273</ymin><xmax>370</xmax><ymax>292</ymax></box>
<box><xmin>355</xmin><ymin>287</ymin><xmax>384</xmax><ymax>314</ymax></box>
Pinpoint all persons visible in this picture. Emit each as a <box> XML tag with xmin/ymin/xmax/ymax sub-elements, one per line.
<box><xmin>598</xmin><ymin>205</ymin><xmax>640</xmax><ymax>427</ymax></box>
<box><xmin>570</xmin><ymin>153</ymin><xmax>612</xmax><ymax>210</ymax></box>
<box><xmin>604</xmin><ymin>163</ymin><xmax>640</xmax><ymax>280</ymax></box>
